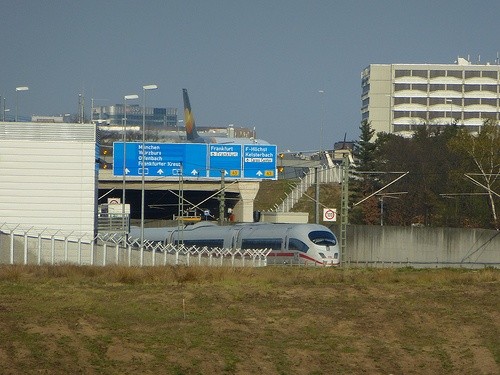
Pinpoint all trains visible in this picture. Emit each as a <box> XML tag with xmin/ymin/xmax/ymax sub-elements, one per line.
<box><xmin>99</xmin><ymin>221</ymin><xmax>340</xmax><ymax>270</ymax></box>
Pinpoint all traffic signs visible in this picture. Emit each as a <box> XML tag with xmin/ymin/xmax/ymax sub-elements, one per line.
<box><xmin>112</xmin><ymin>142</ymin><xmax>277</xmax><ymax>181</ymax></box>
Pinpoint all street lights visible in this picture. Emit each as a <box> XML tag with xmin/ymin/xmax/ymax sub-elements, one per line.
<box><xmin>141</xmin><ymin>84</ymin><xmax>159</xmax><ymax>267</ymax></box>
<box><xmin>15</xmin><ymin>87</ymin><xmax>30</xmax><ymax>120</ymax></box>
<box><xmin>120</xmin><ymin>95</ymin><xmax>140</xmax><ymax>248</ymax></box>
